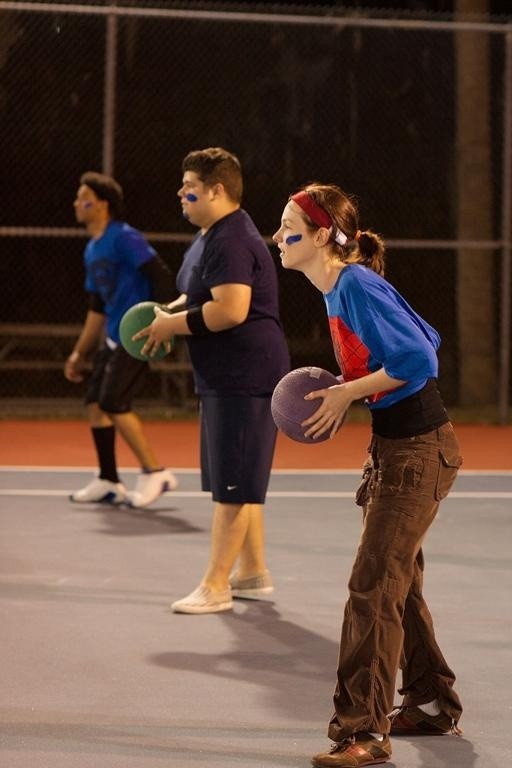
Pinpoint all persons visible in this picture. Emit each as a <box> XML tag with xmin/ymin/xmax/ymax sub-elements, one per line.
<box><xmin>132</xmin><ymin>147</ymin><xmax>291</xmax><ymax>614</ymax></box>
<box><xmin>272</xmin><ymin>183</ymin><xmax>463</xmax><ymax>768</ymax></box>
<box><xmin>64</xmin><ymin>171</ymin><xmax>178</xmax><ymax>509</ymax></box>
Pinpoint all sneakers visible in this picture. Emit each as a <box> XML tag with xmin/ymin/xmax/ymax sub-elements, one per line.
<box><xmin>128</xmin><ymin>469</ymin><xmax>178</xmax><ymax>508</ymax></box>
<box><xmin>386</xmin><ymin>702</ymin><xmax>453</xmax><ymax>734</ymax></box>
<box><xmin>229</xmin><ymin>569</ymin><xmax>275</xmax><ymax>599</ymax></box>
<box><xmin>68</xmin><ymin>476</ymin><xmax>126</xmax><ymax>504</ymax></box>
<box><xmin>172</xmin><ymin>583</ymin><xmax>233</xmax><ymax>613</ymax></box>
<box><xmin>310</xmin><ymin>732</ymin><xmax>393</xmax><ymax>767</ymax></box>
